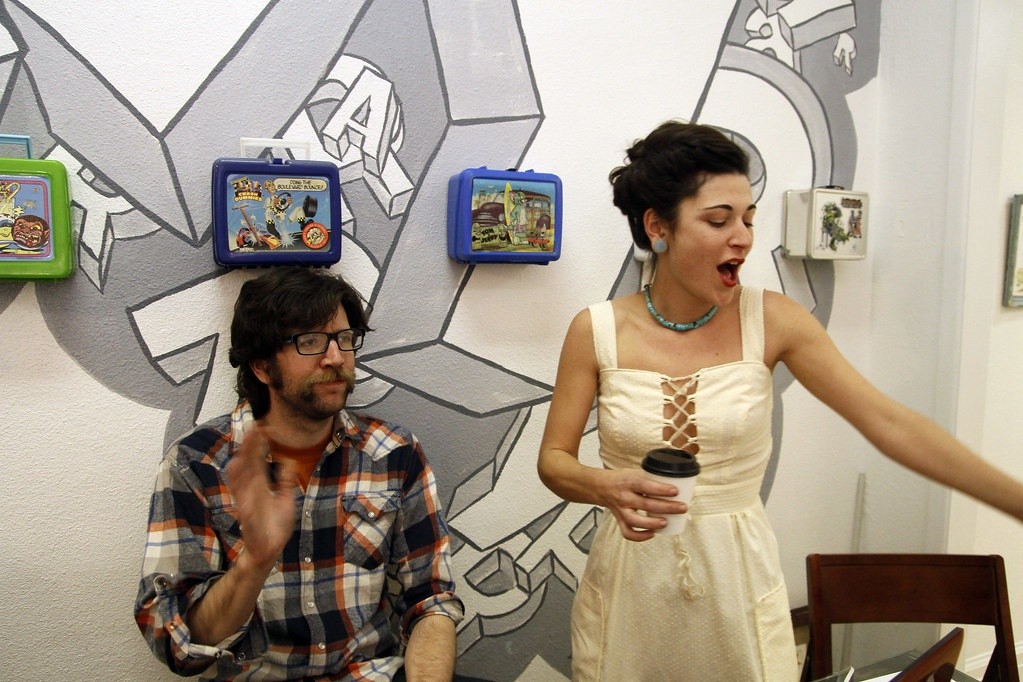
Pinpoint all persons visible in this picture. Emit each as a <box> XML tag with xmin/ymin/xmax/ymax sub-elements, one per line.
<box><xmin>134</xmin><ymin>263</ymin><xmax>491</xmax><ymax>682</ymax></box>
<box><xmin>537</xmin><ymin>118</ymin><xmax>1023</xmax><ymax>681</ymax></box>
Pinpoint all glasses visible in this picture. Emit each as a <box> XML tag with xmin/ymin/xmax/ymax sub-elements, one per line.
<box><xmin>286</xmin><ymin>328</ymin><xmax>365</xmax><ymax>355</ymax></box>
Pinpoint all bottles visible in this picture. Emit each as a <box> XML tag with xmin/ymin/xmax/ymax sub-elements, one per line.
<box><xmin>642</xmin><ymin>447</ymin><xmax>701</xmax><ymax>536</ymax></box>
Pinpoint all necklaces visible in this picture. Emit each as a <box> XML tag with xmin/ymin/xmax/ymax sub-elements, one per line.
<box><xmin>644</xmin><ymin>283</ymin><xmax>718</xmax><ymax>331</ymax></box>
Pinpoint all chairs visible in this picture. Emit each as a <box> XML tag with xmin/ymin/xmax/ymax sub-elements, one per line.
<box><xmin>800</xmin><ymin>552</ymin><xmax>1022</xmax><ymax>682</ymax></box>
